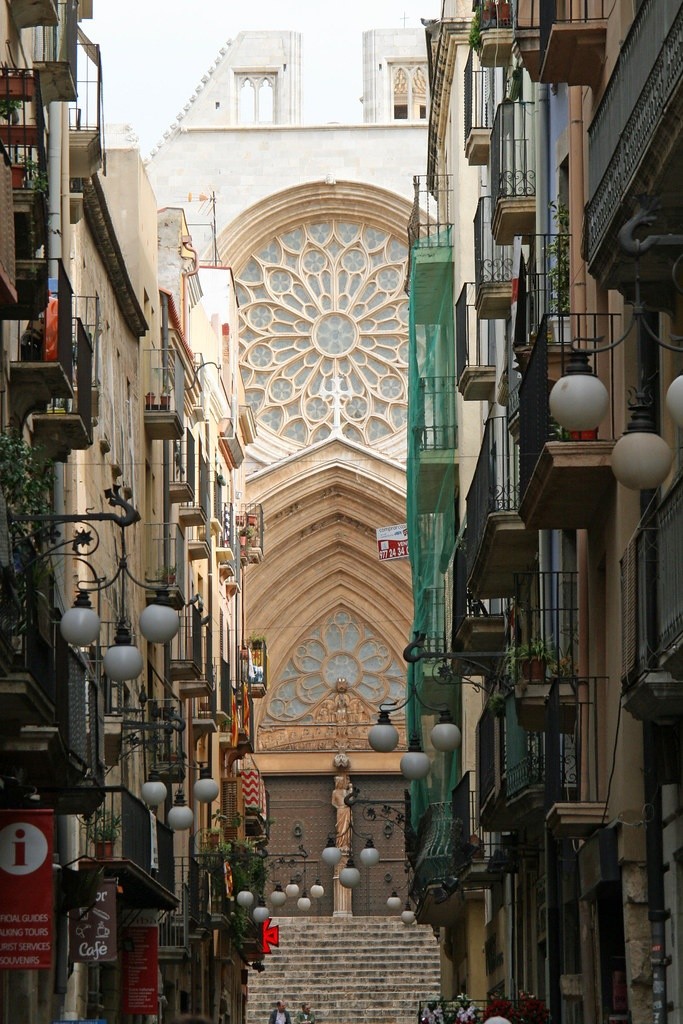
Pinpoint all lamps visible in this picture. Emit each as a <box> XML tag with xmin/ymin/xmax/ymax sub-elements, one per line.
<box><xmin>386</xmin><ymin>860</ymin><xmax>416</xmax><ymax>924</ymax></box>
<box><xmin>548</xmin><ymin>194</ymin><xmax>683</xmax><ymax>489</ymax></box>
<box><xmin>367</xmin><ymin>631</ymin><xmax>513</xmax><ymax>782</ymax></box>
<box><xmin>194</xmin><ymin>846</ymin><xmax>324</xmax><ymax>922</ymax></box>
<box><xmin>7</xmin><ymin>485</ymin><xmax>180</xmax><ymax>681</ymax></box>
<box><xmin>321</xmin><ymin>782</ymin><xmax>418</xmax><ymax>889</ymax></box>
<box><xmin>123</xmin><ymin>706</ymin><xmax>219</xmax><ymax>832</ymax></box>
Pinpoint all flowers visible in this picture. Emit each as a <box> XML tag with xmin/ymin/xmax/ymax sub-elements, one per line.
<box><xmin>419</xmin><ymin>992</ymin><xmax>549</xmax><ymax>1024</ymax></box>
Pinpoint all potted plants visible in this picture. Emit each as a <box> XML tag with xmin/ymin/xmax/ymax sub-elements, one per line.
<box><xmin>0</xmin><ymin>76</ymin><xmax>32</xmax><ymax>120</ymax></box>
<box><xmin>219</xmin><ymin>719</ymin><xmax>232</xmax><ymax>733</ymax></box>
<box><xmin>86</xmin><ymin>808</ymin><xmax>121</xmax><ymax>860</ymax></box>
<box><xmin>145</xmin><ymin>392</ymin><xmax>156</xmax><ymax>405</ymax></box>
<box><xmin>160</xmin><ymin>384</ymin><xmax>174</xmax><ymax>405</ymax></box>
<box><xmin>12</xmin><ymin>154</ymin><xmax>49</xmax><ymax>195</ymax></box>
<box><xmin>549</xmin><ymin>416</ymin><xmax>599</xmax><ymax>441</ymax></box>
<box><xmin>548</xmin><ymin>200</ymin><xmax>570</xmax><ymax>343</ymax></box>
<box><xmin>154</xmin><ymin>562</ymin><xmax>177</xmax><ymax>584</ymax></box>
<box><xmin>505</xmin><ymin>639</ymin><xmax>557</xmax><ymax>680</ymax></box>
<box><xmin>149</xmin><ymin>729</ymin><xmax>178</xmax><ymax>762</ymax></box>
<box><xmin>239</xmin><ymin>527</ymin><xmax>254</xmax><ymax>545</ymax></box>
<box><xmin>243</xmin><ymin>630</ymin><xmax>265</xmax><ymax>649</ymax></box>
<box><xmin>469</xmin><ymin>0</ymin><xmax>513</xmax><ymax>51</ymax></box>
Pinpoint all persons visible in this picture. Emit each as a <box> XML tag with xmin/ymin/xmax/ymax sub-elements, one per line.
<box><xmin>293</xmin><ymin>1003</ymin><xmax>316</xmax><ymax>1024</ymax></box>
<box><xmin>330</xmin><ymin>776</ymin><xmax>354</xmax><ymax>852</ymax></box>
<box><xmin>268</xmin><ymin>1000</ymin><xmax>292</xmax><ymax>1024</ymax></box>
